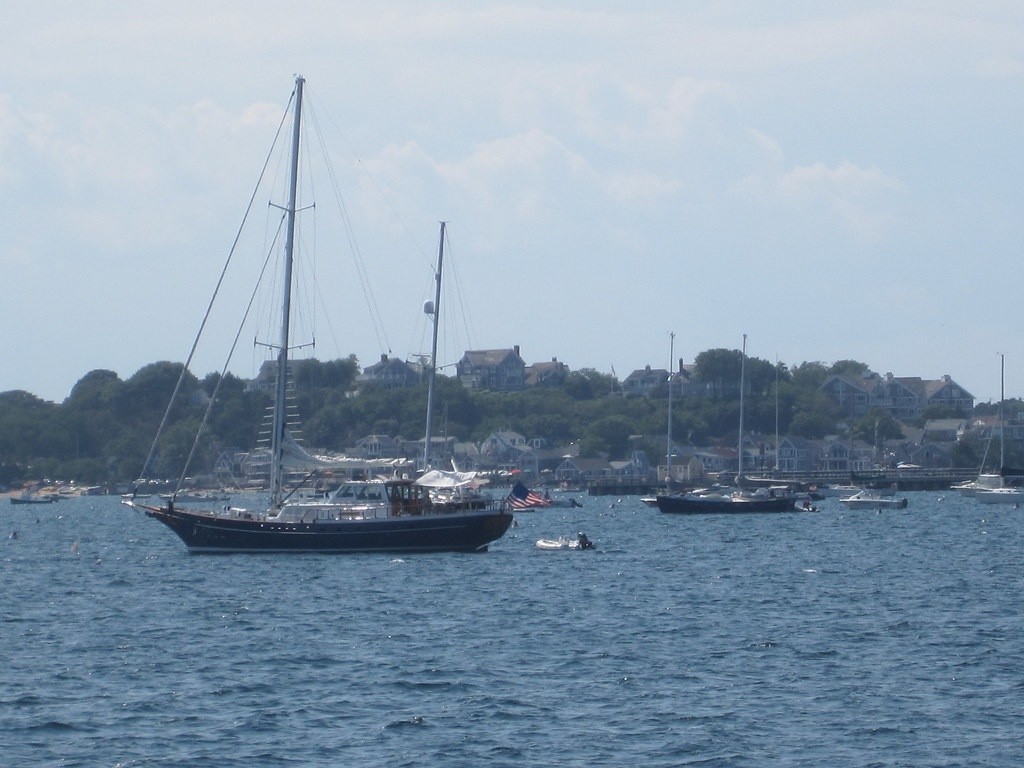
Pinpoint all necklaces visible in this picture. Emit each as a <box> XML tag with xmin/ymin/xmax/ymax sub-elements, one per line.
<box><xmin>803</xmin><ymin>498</ymin><xmax>812</xmax><ymax>511</ymax></box>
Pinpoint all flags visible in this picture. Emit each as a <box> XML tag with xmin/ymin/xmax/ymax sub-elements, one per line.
<box><xmin>503</xmin><ymin>481</ymin><xmax>551</xmax><ymax>511</ymax></box>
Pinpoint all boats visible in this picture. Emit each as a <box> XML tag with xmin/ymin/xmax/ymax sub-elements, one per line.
<box><xmin>157</xmin><ymin>490</ymin><xmax>230</xmax><ymax>502</ymax></box>
<box><xmin>121</xmin><ymin>494</ymin><xmax>152</xmax><ymax>500</ymax></box>
<box><xmin>527</xmin><ymin>489</ymin><xmax>576</xmax><ymax>508</ymax></box>
<box><xmin>948</xmin><ymin>353</ymin><xmax>1011</xmax><ymax>497</ymax></box>
<box><xmin>535</xmin><ymin>538</ymin><xmax>583</xmax><ymax>550</ymax></box>
<box><xmin>655</xmin><ymin>333</ymin><xmax>799</xmax><ymax>513</ymax></box>
<box><xmin>125</xmin><ymin>76</ymin><xmax>513</xmax><ymax>554</ymax></box>
<box><xmin>734</xmin><ymin>333</ymin><xmax>863</xmax><ymax>500</ymax></box>
<box><xmin>976</xmin><ymin>483</ymin><xmax>1024</xmax><ymax>505</ymax></box>
<box><xmin>553</xmin><ymin>485</ymin><xmax>581</xmax><ymax>494</ymax></box>
<box><xmin>9</xmin><ymin>494</ymin><xmax>59</xmax><ymax>506</ymax></box>
<box><xmin>642</xmin><ymin>333</ymin><xmax>731</xmax><ymax>509</ymax></box>
<box><xmin>839</xmin><ymin>489</ymin><xmax>908</xmax><ymax>510</ymax></box>
